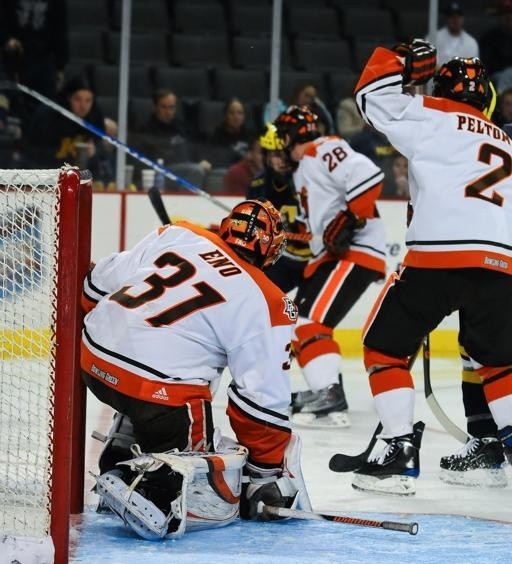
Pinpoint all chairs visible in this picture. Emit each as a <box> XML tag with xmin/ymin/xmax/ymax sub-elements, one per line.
<box><xmin>398</xmin><ymin>12</ymin><xmax>450</xmax><ymax>41</ymax></box>
<box><xmin>334</xmin><ymin>75</ymin><xmax>361</xmax><ymax>109</ymax></box>
<box><xmin>172</xmin><ymin>1</ymin><xmax>227</xmax><ymax>36</ymax></box>
<box><xmin>295</xmin><ymin>38</ymin><xmax>353</xmax><ymax>72</ymax></box>
<box><xmin>213</xmin><ymin>68</ymin><xmax>268</xmax><ymax>104</ymax></box>
<box><xmin>278</xmin><ymin>73</ymin><xmax>329</xmax><ymax>108</ymax></box>
<box><xmin>129</xmin><ymin>99</ymin><xmax>186</xmax><ymax>131</ymax></box>
<box><xmin>197</xmin><ymin>101</ymin><xmax>256</xmax><ymax>137</ymax></box>
<box><xmin>287</xmin><ymin>3</ymin><xmax>339</xmax><ymax>40</ymax></box>
<box><xmin>171</xmin><ymin>35</ymin><xmax>232</xmax><ymax>68</ymax></box>
<box><xmin>342</xmin><ymin>7</ymin><xmax>395</xmax><ymax>41</ymax></box>
<box><xmin>66</xmin><ymin>0</ymin><xmax>113</xmax><ymax>29</ymax></box>
<box><xmin>128</xmin><ymin>33</ymin><xmax>168</xmax><ymax>67</ymax></box>
<box><xmin>95</xmin><ymin>98</ymin><xmax>118</xmax><ymax>128</ymax></box>
<box><xmin>80</xmin><ymin>66</ymin><xmax>125</xmax><ymax>97</ymax></box>
<box><xmin>230</xmin><ymin>0</ymin><xmax>273</xmax><ymax>37</ymax></box>
<box><xmin>65</xmin><ymin>31</ymin><xmax>113</xmax><ymax>65</ymax></box>
<box><xmin>114</xmin><ymin>0</ymin><xmax>169</xmax><ymax>34</ymax></box>
<box><xmin>234</xmin><ymin>37</ymin><xmax>289</xmax><ymax>69</ymax></box>
<box><xmin>155</xmin><ymin>67</ymin><xmax>210</xmax><ymax>103</ymax></box>
<box><xmin>355</xmin><ymin>42</ymin><xmax>407</xmax><ymax>72</ymax></box>
<box><xmin>128</xmin><ymin>65</ymin><xmax>154</xmax><ymax>99</ymax></box>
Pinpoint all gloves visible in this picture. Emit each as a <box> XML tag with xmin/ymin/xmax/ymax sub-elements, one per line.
<box><xmin>238</xmin><ymin>460</ymin><xmax>298</xmax><ymax>522</ymax></box>
<box><xmin>391</xmin><ymin>38</ymin><xmax>437</xmax><ymax>87</ymax></box>
<box><xmin>323</xmin><ymin>209</ymin><xmax>367</xmax><ymax>262</ymax></box>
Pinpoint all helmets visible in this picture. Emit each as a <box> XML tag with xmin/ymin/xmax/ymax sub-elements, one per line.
<box><xmin>274</xmin><ymin>104</ymin><xmax>322</xmax><ymax>156</ymax></box>
<box><xmin>217</xmin><ymin>196</ymin><xmax>288</xmax><ymax>269</ymax></box>
<box><xmin>431</xmin><ymin>56</ymin><xmax>493</xmax><ymax>116</ymax></box>
<box><xmin>258</xmin><ymin>122</ymin><xmax>288</xmax><ymax>151</ymax></box>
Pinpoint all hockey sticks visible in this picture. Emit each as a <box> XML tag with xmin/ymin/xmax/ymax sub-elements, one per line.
<box><xmin>147</xmin><ymin>185</ymin><xmax>173</xmax><ymax>226</ymax></box>
<box><xmin>262</xmin><ymin>504</ymin><xmax>419</xmax><ymax>536</ymax></box>
<box><xmin>423</xmin><ymin>332</ymin><xmax>470</xmax><ymax>445</ymax></box>
<box><xmin>328</xmin><ymin>336</ymin><xmax>426</xmax><ymax>473</ymax></box>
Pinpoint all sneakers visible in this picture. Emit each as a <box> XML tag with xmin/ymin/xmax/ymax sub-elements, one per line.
<box><xmin>438</xmin><ymin>430</ymin><xmax>508</xmax><ymax>488</ymax></box>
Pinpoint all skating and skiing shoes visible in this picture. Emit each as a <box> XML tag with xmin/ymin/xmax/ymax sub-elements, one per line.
<box><xmin>352</xmin><ymin>421</ymin><xmax>425</xmax><ymax>496</ymax></box>
<box><xmin>88</xmin><ymin>444</ymin><xmax>187</xmax><ymax>541</ymax></box>
<box><xmin>289</xmin><ymin>374</ymin><xmax>351</xmax><ymax>430</ymax></box>
<box><xmin>92</xmin><ymin>412</ymin><xmax>137</xmax><ymax>515</ymax></box>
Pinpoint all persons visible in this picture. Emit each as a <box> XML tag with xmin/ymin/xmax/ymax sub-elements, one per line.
<box><xmin>275</xmin><ymin>106</ymin><xmax>388</xmax><ymax>426</ymax></box>
<box><xmin>349</xmin><ymin>37</ymin><xmax>510</xmax><ymax>492</ymax></box>
<box><xmin>424</xmin><ymin>3</ymin><xmax>478</xmax><ymax>97</ymax></box>
<box><xmin>246</xmin><ymin>124</ymin><xmax>312</xmax><ymax>292</ymax></box>
<box><xmin>42</xmin><ymin>80</ymin><xmax>118</xmax><ymax>184</ymax></box>
<box><xmin>132</xmin><ymin>90</ymin><xmax>210</xmax><ymax>171</ymax></box>
<box><xmin>338</xmin><ymin>75</ymin><xmax>366</xmax><ymax>135</ymax></box>
<box><xmin>381</xmin><ymin>148</ymin><xmax>409</xmax><ymax>197</ymax></box>
<box><xmin>286</xmin><ymin>86</ymin><xmax>332</xmax><ymax>135</ymax></box>
<box><xmin>80</xmin><ymin>197</ymin><xmax>299</xmax><ymax>543</ymax></box>
<box><xmin>213</xmin><ymin>99</ymin><xmax>252</xmax><ymax>166</ymax></box>
